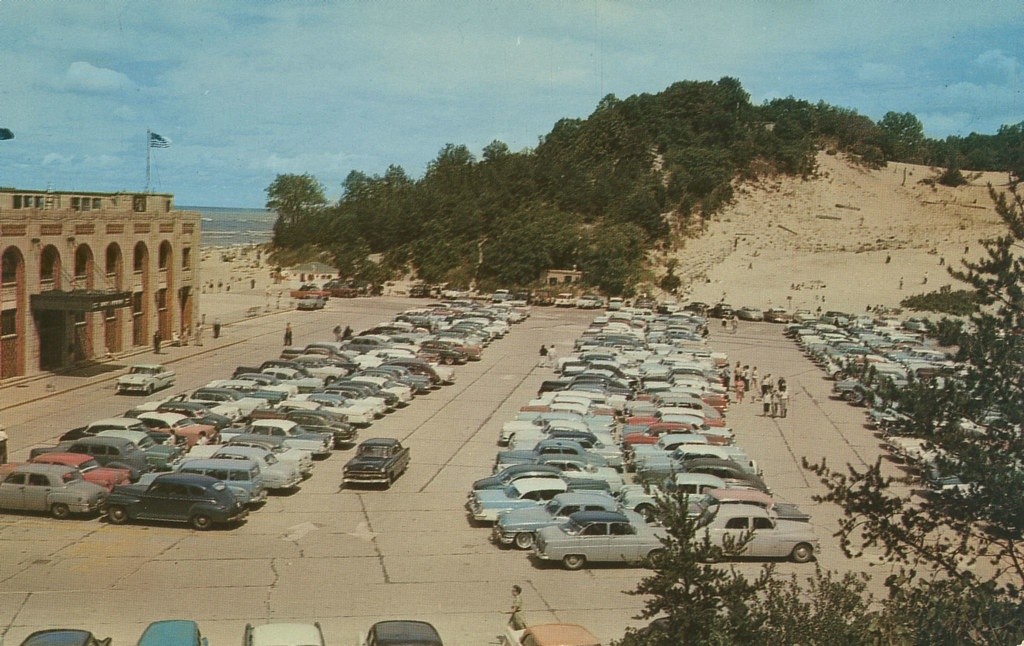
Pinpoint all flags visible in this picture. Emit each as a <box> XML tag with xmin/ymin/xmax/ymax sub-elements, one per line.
<box><xmin>151</xmin><ymin>133</ymin><xmax>171</xmax><ymax>148</ymax></box>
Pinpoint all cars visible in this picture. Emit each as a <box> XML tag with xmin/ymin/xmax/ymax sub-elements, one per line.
<box><xmin>323</xmin><ymin>279</ymin><xmax>384</xmax><ymax>297</ymax></box>
<box><xmin>362</xmin><ymin>620</ymin><xmax>443</xmax><ymax>646</ymax></box>
<box><xmin>115</xmin><ymin>365</ymin><xmax>176</xmax><ymax>395</ymax></box>
<box><xmin>290</xmin><ymin>285</ymin><xmax>331</xmax><ymax>300</ymax></box>
<box><xmin>784</xmin><ymin>309</ymin><xmax>1022</xmax><ymax>520</ymax></box>
<box><xmin>410</xmin><ymin>283</ymin><xmax>471</xmax><ymax>300</ymax></box>
<box><xmin>342</xmin><ymin>438</ymin><xmax>412</xmax><ymax>488</ymax></box>
<box><xmin>298</xmin><ymin>294</ymin><xmax>326</xmax><ymax>310</ymax></box>
<box><xmin>17</xmin><ymin>628</ymin><xmax>111</xmax><ymax>645</ymax></box>
<box><xmin>1</xmin><ymin>288</ymin><xmax>603</xmax><ymax>531</ymax></box>
<box><xmin>136</xmin><ymin>620</ymin><xmax>208</xmax><ymax>646</ymax></box>
<box><xmin>467</xmin><ymin>298</ymin><xmax>821</xmax><ymax>570</ymax></box>
<box><xmin>243</xmin><ymin>620</ymin><xmax>327</xmax><ymax>645</ymax></box>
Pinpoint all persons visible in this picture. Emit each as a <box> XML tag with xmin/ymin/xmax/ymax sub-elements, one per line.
<box><xmin>208</xmin><ymin>427</ymin><xmax>219</xmax><ymax>440</ymax></box>
<box><xmin>213</xmin><ymin>317</ymin><xmax>220</xmax><ymax>337</ymax></box>
<box><xmin>722</xmin><ymin>361</ymin><xmax>758</xmax><ymax>404</ymax></box>
<box><xmin>154</xmin><ymin>330</ymin><xmax>161</xmax><ymax>354</ymax></box>
<box><xmin>886</xmin><ymin>251</ymin><xmax>890</xmax><ymax>265</ymax></box>
<box><xmin>251</xmin><ymin>279</ymin><xmax>255</xmax><ymax>288</ymax></box>
<box><xmin>284</xmin><ymin>323</ymin><xmax>292</xmax><ymax>345</ymax></box>
<box><xmin>548</xmin><ymin>345</ymin><xmax>557</xmax><ymax>363</ymax></box>
<box><xmin>498</xmin><ymin>584</ymin><xmax>528</xmax><ymax>646</ymax></box>
<box><xmin>196</xmin><ymin>431</ymin><xmax>209</xmax><ymax>444</ymax></box>
<box><xmin>722</xmin><ymin>314</ymin><xmax>738</xmax><ymax>329</ymax></box>
<box><xmin>539</xmin><ymin>344</ymin><xmax>548</xmax><ymax>367</ymax></box>
<box><xmin>334</xmin><ymin>325</ymin><xmax>353</xmax><ymax>342</ymax></box>
<box><xmin>163</xmin><ymin>429</ymin><xmax>176</xmax><ymax>444</ymax></box>
<box><xmin>928</xmin><ymin>247</ymin><xmax>944</xmax><ymax>265</ymax></box>
<box><xmin>699</xmin><ymin>387</ymin><xmax>707</xmax><ymax>395</ymax></box>
<box><xmin>701</xmin><ymin>327</ymin><xmax>709</xmax><ymax>338</ymax></box>
<box><xmin>172</xmin><ymin>321</ymin><xmax>205</xmax><ymax>347</ymax></box>
<box><xmin>751</xmin><ymin>374</ymin><xmax>789</xmax><ymax>418</ymax></box>
<box><xmin>923</xmin><ymin>271</ymin><xmax>928</xmax><ymax>283</ymax></box>
<box><xmin>899</xmin><ymin>277</ymin><xmax>904</xmax><ymax>289</ymax></box>
<box><xmin>965</xmin><ymin>244</ymin><xmax>970</xmax><ymax>253</ymax></box>
